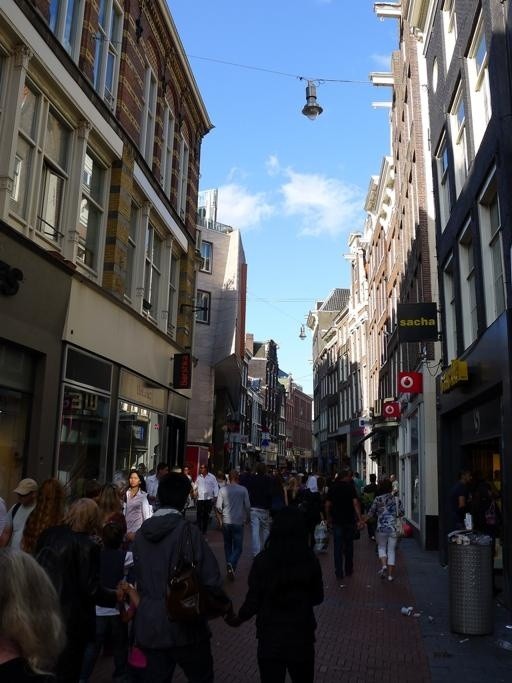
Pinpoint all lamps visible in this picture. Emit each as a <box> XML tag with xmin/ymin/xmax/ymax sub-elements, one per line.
<box><xmin>298</xmin><ymin>324</ymin><xmax>307</xmax><ymax>340</ymax></box>
<box><xmin>302</xmin><ymin>80</ymin><xmax>323</xmax><ymax>120</ymax></box>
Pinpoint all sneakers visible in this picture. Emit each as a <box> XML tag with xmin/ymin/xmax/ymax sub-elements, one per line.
<box><xmin>379</xmin><ymin>565</ymin><xmax>388</xmax><ymax>578</ymax></box>
<box><xmin>226</xmin><ymin>562</ymin><xmax>236</xmax><ymax>581</ymax></box>
<box><xmin>387</xmin><ymin>574</ymin><xmax>394</xmax><ymax>581</ymax></box>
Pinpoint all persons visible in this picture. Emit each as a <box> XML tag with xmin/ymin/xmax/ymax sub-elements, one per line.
<box><xmin>3</xmin><ymin>477</ymin><xmax>130</xmax><ymax>658</ymax></box>
<box><xmin>122</xmin><ymin>462</ymin><xmax>404</xmax><ymax>580</ymax></box>
<box><xmin>224</xmin><ymin>505</ymin><xmax>324</xmax><ymax>681</ymax></box>
<box><xmin>34</xmin><ymin>497</ymin><xmax>122</xmax><ymax>681</ymax></box>
<box><xmin>117</xmin><ymin>472</ymin><xmax>236</xmax><ymax>680</ymax></box>
<box><xmin>79</xmin><ymin>523</ymin><xmax>130</xmax><ymax>681</ymax></box>
<box><xmin>1</xmin><ymin>545</ymin><xmax>65</xmax><ymax>681</ymax></box>
<box><xmin>448</xmin><ymin>468</ymin><xmax>473</xmax><ymax>529</ymax></box>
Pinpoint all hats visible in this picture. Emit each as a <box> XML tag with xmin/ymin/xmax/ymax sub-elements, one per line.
<box><xmin>13</xmin><ymin>479</ymin><xmax>38</xmax><ymax>496</ymax></box>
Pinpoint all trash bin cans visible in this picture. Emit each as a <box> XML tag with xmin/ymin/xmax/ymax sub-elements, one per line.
<box><xmin>447</xmin><ymin>530</ymin><xmax>494</xmax><ymax>633</ymax></box>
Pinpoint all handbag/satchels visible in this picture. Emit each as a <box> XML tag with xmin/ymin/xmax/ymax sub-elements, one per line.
<box><xmin>314</xmin><ymin>524</ymin><xmax>329</xmax><ymax>553</ymax></box>
<box><xmin>166</xmin><ymin>560</ymin><xmax>223</xmax><ymax>620</ymax></box>
<box><xmin>396</xmin><ymin>518</ymin><xmax>405</xmax><ymax>537</ymax></box>
